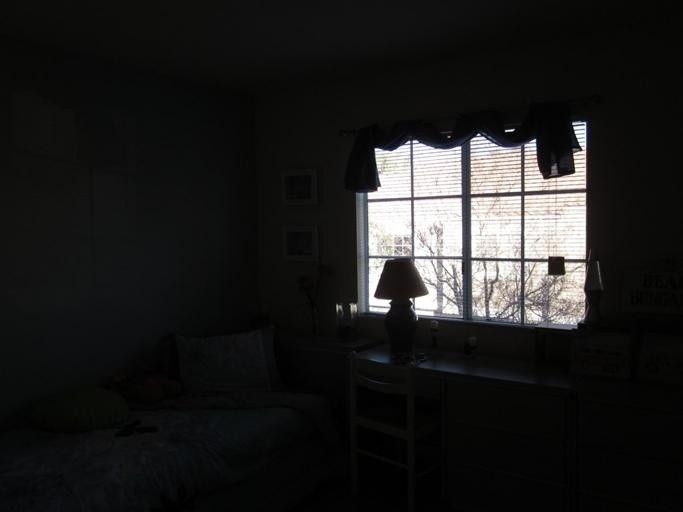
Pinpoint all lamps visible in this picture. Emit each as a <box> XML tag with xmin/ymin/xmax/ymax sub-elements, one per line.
<box><xmin>371</xmin><ymin>256</ymin><xmax>429</xmax><ymax>356</ymax></box>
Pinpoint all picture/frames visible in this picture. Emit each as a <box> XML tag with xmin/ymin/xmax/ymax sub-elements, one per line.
<box><xmin>277</xmin><ymin>164</ymin><xmax>320</xmax><ymax>209</ymax></box>
<box><xmin>280</xmin><ymin>222</ymin><xmax>323</xmax><ymax>265</ymax></box>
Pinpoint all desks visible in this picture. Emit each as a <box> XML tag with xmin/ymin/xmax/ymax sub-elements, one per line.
<box><xmin>348</xmin><ymin>342</ymin><xmax>596</xmax><ymax>512</ymax></box>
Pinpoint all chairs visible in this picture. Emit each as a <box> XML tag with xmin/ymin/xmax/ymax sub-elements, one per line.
<box><xmin>350</xmin><ymin>357</ymin><xmax>445</xmax><ymax>512</ymax></box>
